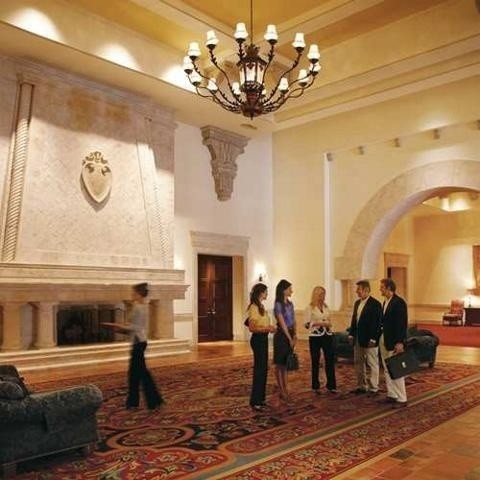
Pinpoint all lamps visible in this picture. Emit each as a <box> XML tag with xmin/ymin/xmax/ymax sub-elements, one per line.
<box><xmin>181</xmin><ymin>1</ymin><xmax>324</xmax><ymax>119</ymax></box>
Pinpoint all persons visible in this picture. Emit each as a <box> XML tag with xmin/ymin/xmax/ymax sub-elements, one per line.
<box><xmin>273</xmin><ymin>279</ymin><xmax>298</xmax><ymax>404</ymax></box>
<box><xmin>245</xmin><ymin>283</ymin><xmax>278</xmax><ymax>412</ymax></box>
<box><xmin>301</xmin><ymin>285</ymin><xmax>341</xmax><ymax>396</ymax></box>
<box><xmin>375</xmin><ymin>278</ymin><xmax>410</xmax><ymax>409</ymax></box>
<box><xmin>346</xmin><ymin>279</ymin><xmax>381</xmax><ymax>400</ymax></box>
<box><xmin>100</xmin><ymin>282</ymin><xmax>169</xmax><ymax>415</ymax></box>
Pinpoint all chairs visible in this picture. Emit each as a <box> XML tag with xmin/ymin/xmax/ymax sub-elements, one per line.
<box><xmin>0</xmin><ymin>366</ymin><xmax>102</xmax><ymax>480</ymax></box>
<box><xmin>331</xmin><ymin>322</ymin><xmax>439</xmax><ymax>370</ymax></box>
<box><xmin>442</xmin><ymin>299</ymin><xmax>464</xmax><ymax>326</ymax></box>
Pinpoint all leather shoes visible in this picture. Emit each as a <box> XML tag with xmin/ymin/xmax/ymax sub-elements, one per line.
<box><xmin>376</xmin><ymin>395</ymin><xmax>407</xmax><ymax>408</ymax></box>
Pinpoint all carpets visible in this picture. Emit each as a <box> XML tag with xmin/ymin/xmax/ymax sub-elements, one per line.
<box><xmin>0</xmin><ymin>350</ymin><xmax>480</xmax><ymax>480</ymax></box>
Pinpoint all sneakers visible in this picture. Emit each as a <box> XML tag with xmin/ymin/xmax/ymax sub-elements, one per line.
<box><xmin>350</xmin><ymin>386</ymin><xmax>379</xmax><ymax>397</ymax></box>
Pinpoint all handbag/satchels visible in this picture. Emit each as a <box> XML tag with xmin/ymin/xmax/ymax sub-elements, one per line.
<box><xmin>277</xmin><ymin>346</ymin><xmax>299</xmax><ymax>372</ymax></box>
<box><xmin>384</xmin><ymin>349</ymin><xmax>421</xmax><ymax>380</ymax></box>
